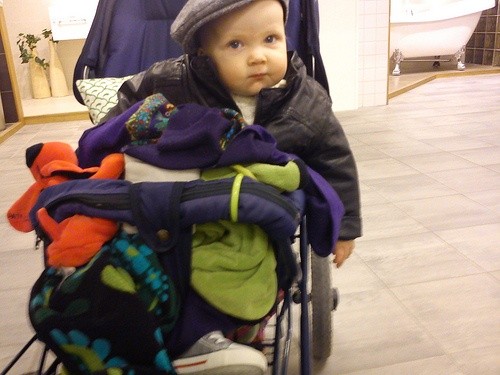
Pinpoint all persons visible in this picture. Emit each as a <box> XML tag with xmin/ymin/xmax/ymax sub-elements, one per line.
<box><xmin>95</xmin><ymin>0</ymin><xmax>363</xmax><ymax>375</ymax></box>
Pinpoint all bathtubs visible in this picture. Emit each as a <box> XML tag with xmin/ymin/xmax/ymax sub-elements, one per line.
<box><xmin>390</xmin><ymin>0</ymin><xmax>496</xmax><ymax>58</ymax></box>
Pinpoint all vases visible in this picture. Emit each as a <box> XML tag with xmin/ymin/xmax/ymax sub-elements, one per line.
<box><xmin>29</xmin><ymin>40</ymin><xmax>69</xmax><ymax>99</ymax></box>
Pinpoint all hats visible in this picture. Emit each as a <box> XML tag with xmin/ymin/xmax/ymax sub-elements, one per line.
<box><xmin>170</xmin><ymin>0</ymin><xmax>288</xmax><ymax>54</ymax></box>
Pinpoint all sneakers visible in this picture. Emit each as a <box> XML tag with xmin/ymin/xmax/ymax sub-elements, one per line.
<box><xmin>173</xmin><ymin>330</ymin><xmax>267</xmax><ymax>374</ymax></box>
